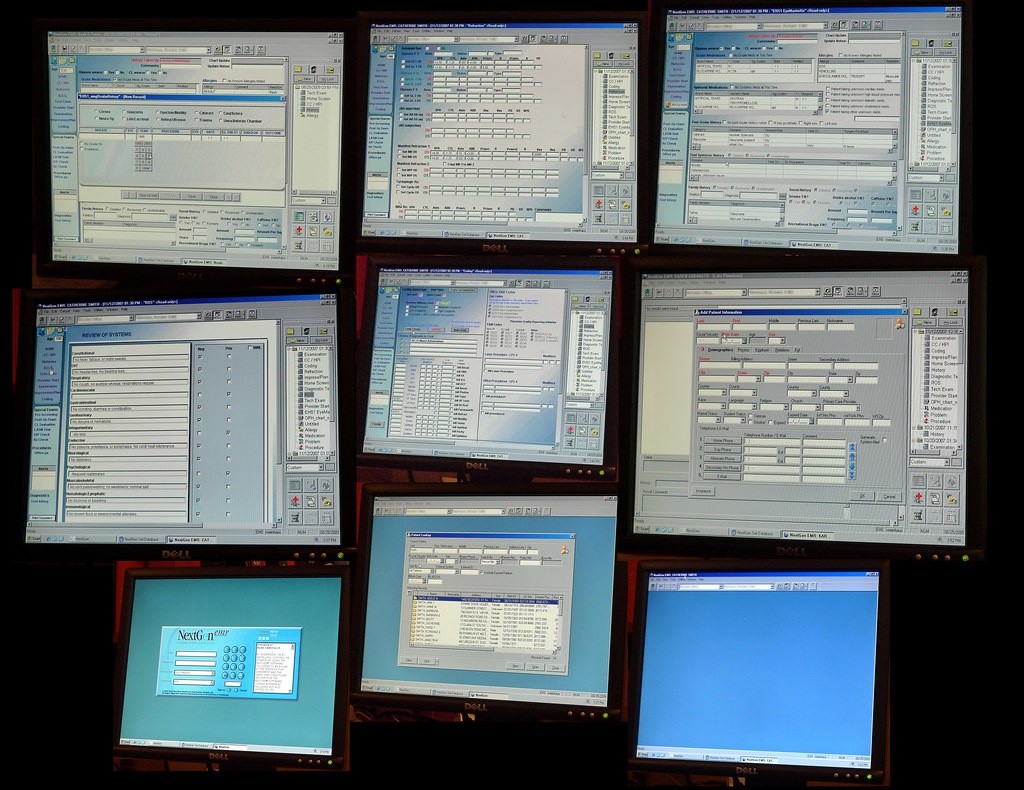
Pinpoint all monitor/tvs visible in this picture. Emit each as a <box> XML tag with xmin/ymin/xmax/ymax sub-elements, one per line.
<box><xmin>17</xmin><ymin>0</ymin><xmax>989</xmax><ymax>790</ymax></box>
